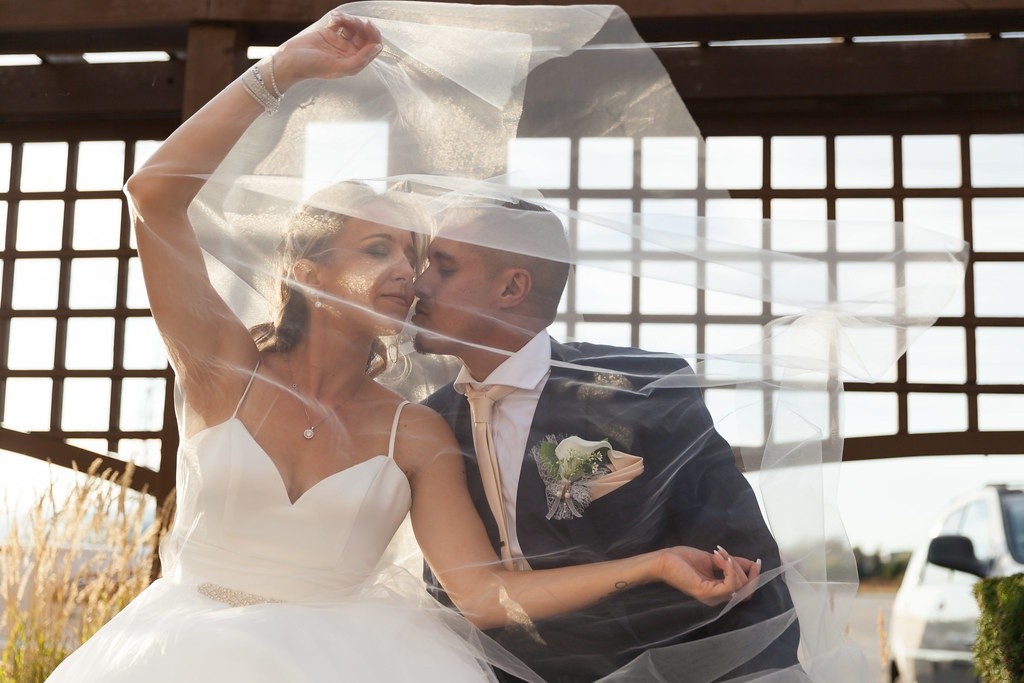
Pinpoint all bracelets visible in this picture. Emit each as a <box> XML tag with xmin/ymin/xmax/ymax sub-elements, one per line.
<box><xmin>240</xmin><ymin>54</ymin><xmax>284</xmax><ymax>114</ymax></box>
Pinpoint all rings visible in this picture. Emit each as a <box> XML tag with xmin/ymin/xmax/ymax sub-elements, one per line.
<box><xmin>728</xmin><ymin>592</ymin><xmax>738</xmax><ymax>600</ymax></box>
<box><xmin>341</xmin><ymin>31</ymin><xmax>350</xmax><ymax>41</ymax></box>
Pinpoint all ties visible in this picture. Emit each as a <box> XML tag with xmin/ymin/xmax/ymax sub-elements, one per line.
<box><xmin>465</xmin><ymin>382</ymin><xmax>518</xmax><ymax>574</ymax></box>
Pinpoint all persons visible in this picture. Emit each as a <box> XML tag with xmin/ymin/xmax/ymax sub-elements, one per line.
<box><xmin>40</xmin><ymin>11</ymin><xmax>762</xmax><ymax>683</ymax></box>
<box><xmin>411</xmin><ymin>197</ymin><xmax>809</xmax><ymax>683</ymax></box>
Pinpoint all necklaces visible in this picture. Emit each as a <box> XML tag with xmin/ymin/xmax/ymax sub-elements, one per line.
<box><xmin>288</xmin><ymin>351</ymin><xmax>366</xmax><ymax>440</ymax></box>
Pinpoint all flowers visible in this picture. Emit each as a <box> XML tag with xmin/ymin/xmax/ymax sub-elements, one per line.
<box><xmin>529</xmin><ymin>431</ymin><xmax>623</xmax><ymax>519</ymax></box>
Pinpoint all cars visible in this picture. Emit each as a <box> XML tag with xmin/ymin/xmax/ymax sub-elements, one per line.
<box><xmin>879</xmin><ymin>482</ymin><xmax>1024</xmax><ymax>683</ymax></box>
